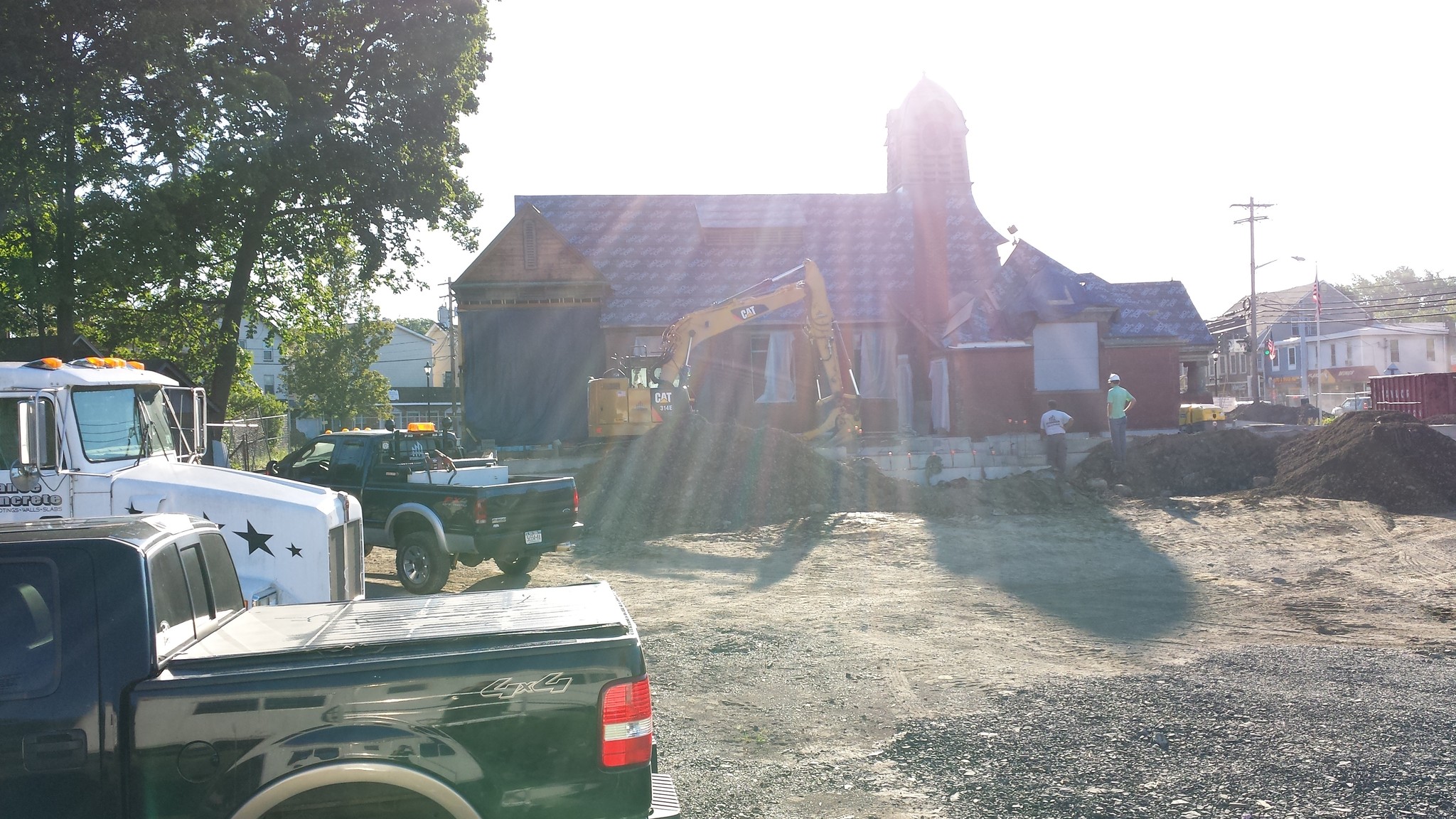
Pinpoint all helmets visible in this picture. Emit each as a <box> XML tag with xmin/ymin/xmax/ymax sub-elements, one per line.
<box><xmin>1109</xmin><ymin>374</ymin><xmax>1120</xmax><ymax>381</ymax></box>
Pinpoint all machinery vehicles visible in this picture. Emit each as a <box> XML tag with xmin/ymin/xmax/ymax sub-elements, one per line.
<box><xmin>587</xmin><ymin>258</ymin><xmax>863</xmax><ymax>458</ymax></box>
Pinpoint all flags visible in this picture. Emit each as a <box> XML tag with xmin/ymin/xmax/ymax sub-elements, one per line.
<box><xmin>1313</xmin><ymin>279</ymin><xmax>1321</xmax><ymax>319</ymax></box>
<box><xmin>1268</xmin><ymin>331</ymin><xmax>1276</xmax><ymax>360</ymax></box>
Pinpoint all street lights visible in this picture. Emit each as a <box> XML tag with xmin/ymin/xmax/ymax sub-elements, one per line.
<box><xmin>1211</xmin><ymin>348</ymin><xmax>1221</xmax><ymax>397</ymax></box>
<box><xmin>1249</xmin><ymin>256</ymin><xmax>1306</xmax><ymax>402</ymax></box>
<box><xmin>423</xmin><ymin>361</ymin><xmax>432</xmax><ymax>423</ymax></box>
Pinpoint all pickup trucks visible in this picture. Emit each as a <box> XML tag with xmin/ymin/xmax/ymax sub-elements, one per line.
<box><xmin>0</xmin><ymin>514</ymin><xmax>681</xmax><ymax>819</ymax></box>
<box><xmin>249</xmin><ymin>420</ymin><xmax>583</xmax><ymax>595</ymax></box>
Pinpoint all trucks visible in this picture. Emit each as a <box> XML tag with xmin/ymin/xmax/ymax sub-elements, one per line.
<box><xmin>1</xmin><ymin>354</ymin><xmax>365</xmax><ymax>607</ymax></box>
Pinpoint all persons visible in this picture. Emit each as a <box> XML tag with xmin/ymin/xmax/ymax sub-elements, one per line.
<box><xmin>1040</xmin><ymin>400</ymin><xmax>1073</xmax><ymax>466</ymax></box>
<box><xmin>1107</xmin><ymin>373</ymin><xmax>1136</xmax><ymax>461</ymax></box>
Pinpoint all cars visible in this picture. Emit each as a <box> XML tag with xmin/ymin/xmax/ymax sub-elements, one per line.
<box><xmin>1331</xmin><ymin>396</ymin><xmax>1372</xmax><ymax>417</ymax></box>
